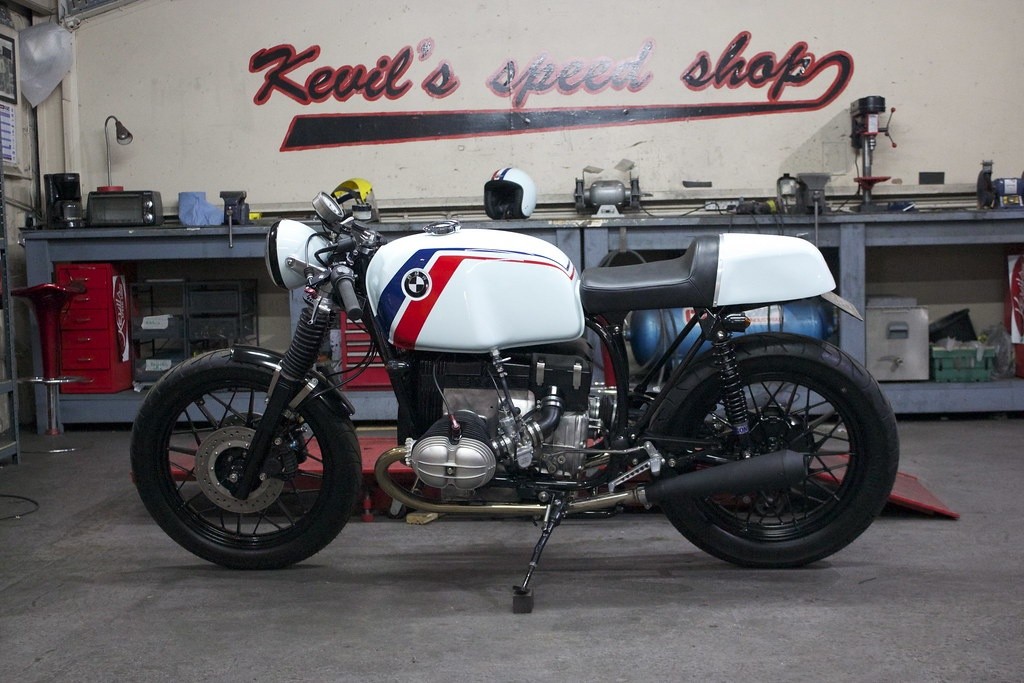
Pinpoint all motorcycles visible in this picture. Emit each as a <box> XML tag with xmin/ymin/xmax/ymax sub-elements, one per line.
<box><xmin>129</xmin><ymin>187</ymin><xmax>902</xmax><ymax>615</ymax></box>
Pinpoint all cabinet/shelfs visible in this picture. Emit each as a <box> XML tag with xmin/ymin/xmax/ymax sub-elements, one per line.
<box><xmin>23</xmin><ymin>203</ymin><xmax>1024</xmax><ymax>436</ymax></box>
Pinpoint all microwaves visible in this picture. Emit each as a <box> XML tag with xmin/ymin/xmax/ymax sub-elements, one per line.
<box><xmin>87</xmin><ymin>190</ymin><xmax>164</xmax><ymax>227</ymax></box>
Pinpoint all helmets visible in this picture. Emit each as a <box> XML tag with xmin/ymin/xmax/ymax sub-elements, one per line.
<box><xmin>484</xmin><ymin>167</ymin><xmax>536</xmax><ymax>218</ymax></box>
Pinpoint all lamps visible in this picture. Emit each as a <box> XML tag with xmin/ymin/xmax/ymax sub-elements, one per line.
<box><xmin>98</xmin><ymin>116</ymin><xmax>132</xmax><ymax>192</ymax></box>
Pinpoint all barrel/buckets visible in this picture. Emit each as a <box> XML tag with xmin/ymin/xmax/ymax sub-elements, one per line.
<box><xmin>929</xmin><ymin>308</ymin><xmax>978</xmax><ymax>342</ymax></box>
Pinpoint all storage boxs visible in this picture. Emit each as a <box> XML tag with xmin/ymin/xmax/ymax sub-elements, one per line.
<box><xmin>864</xmin><ymin>304</ymin><xmax>930</xmax><ymax>382</ymax></box>
<box><xmin>930</xmin><ymin>347</ymin><xmax>994</xmax><ymax>384</ymax></box>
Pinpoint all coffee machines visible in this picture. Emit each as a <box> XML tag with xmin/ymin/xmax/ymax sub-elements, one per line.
<box><xmin>44</xmin><ymin>173</ymin><xmax>85</xmax><ymax>229</ymax></box>
